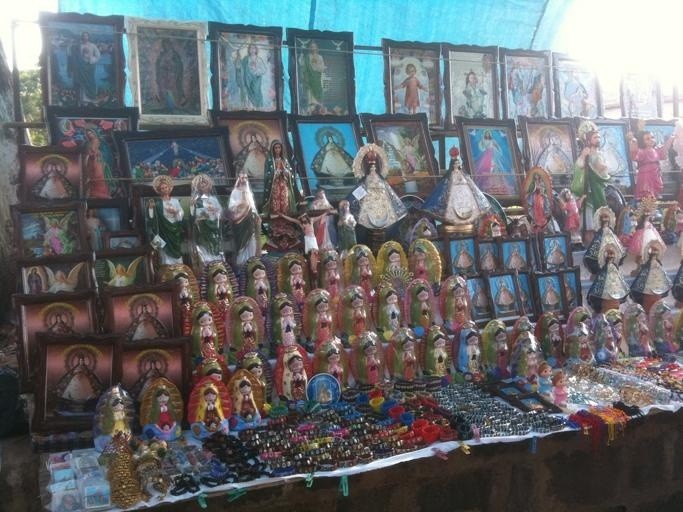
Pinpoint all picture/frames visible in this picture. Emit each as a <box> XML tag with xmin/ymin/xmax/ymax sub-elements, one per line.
<box><xmin>9</xmin><ymin>201</ymin><xmax>193</xmax><ymax>437</ymax></box>
<box><xmin>622</xmin><ymin>66</ymin><xmax>662</xmax><ymax>117</ymax></box>
<box><xmin>383</xmin><ymin>39</ymin><xmax>443</xmax><ymax>130</ymax></box>
<box><xmin>126</xmin><ymin>16</ymin><xmax>209</xmax><ymax>127</ymax></box>
<box><xmin>444</xmin><ymin>234</ymin><xmax>582</xmax><ymax>327</ymax></box>
<box><xmin>287</xmin><ymin>28</ymin><xmax>356</xmax><ymax>121</ymax></box>
<box><xmin>443</xmin><ymin>43</ymin><xmax>500</xmax><ymax>130</ymax></box>
<box><xmin>500</xmin><ymin>48</ymin><xmax>551</xmax><ymax>124</ymax></box>
<box><xmin>4</xmin><ymin>107</ymin><xmax>683</xmax><ymax>201</ymax></box>
<box><xmin>39</xmin><ymin>11</ymin><xmax>126</xmax><ymax>109</ymax></box>
<box><xmin>551</xmin><ymin>52</ymin><xmax>603</xmax><ymax>118</ymax></box>
<box><xmin>208</xmin><ymin>22</ymin><xmax>284</xmax><ymax>116</ymax></box>
